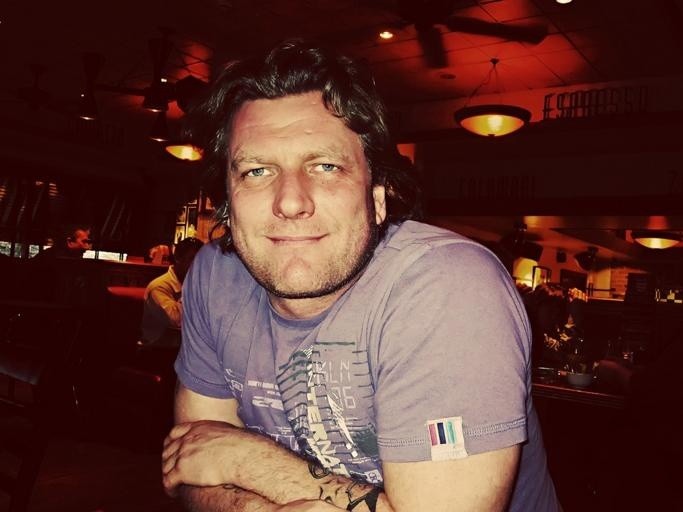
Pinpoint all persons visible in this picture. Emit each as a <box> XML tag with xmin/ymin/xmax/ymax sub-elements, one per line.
<box><xmin>7</xmin><ymin>223</ymin><xmax>161</xmax><ymax>512</ymax></box>
<box><xmin>141</xmin><ymin>237</ymin><xmax>204</xmax><ymax>385</ymax></box>
<box><xmin>591</xmin><ymin>342</ymin><xmax>683</xmax><ymax>498</ymax></box>
<box><xmin>160</xmin><ymin>32</ymin><xmax>565</xmax><ymax>512</ymax></box>
<box><xmin>142</xmin><ymin>239</ymin><xmax>157</xmax><ymax>264</ymax></box>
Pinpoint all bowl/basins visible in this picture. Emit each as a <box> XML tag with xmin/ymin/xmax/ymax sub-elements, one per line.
<box><xmin>567</xmin><ymin>374</ymin><xmax>593</xmax><ymax>387</ymax></box>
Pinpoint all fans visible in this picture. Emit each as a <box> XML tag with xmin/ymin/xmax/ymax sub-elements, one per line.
<box><xmin>341</xmin><ymin>1</ymin><xmax>547</xmax><ymax>70</ymax></box>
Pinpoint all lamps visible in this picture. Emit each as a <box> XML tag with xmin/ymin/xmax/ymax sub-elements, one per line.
<box><xmin>630</xmin><ymin>232</ymin><xmax>681</xmax><ymax>249</ymax></box>
<box><xmin>454</xmin><ymin>58</ymin><xmax>531</xmax><ymax>138</ymax></box>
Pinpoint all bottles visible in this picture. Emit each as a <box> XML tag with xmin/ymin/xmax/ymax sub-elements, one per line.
<box><xmin>654</xmin><ymin>288</ymin><xmax>660</xmax><ymax>302</ymax></box>
